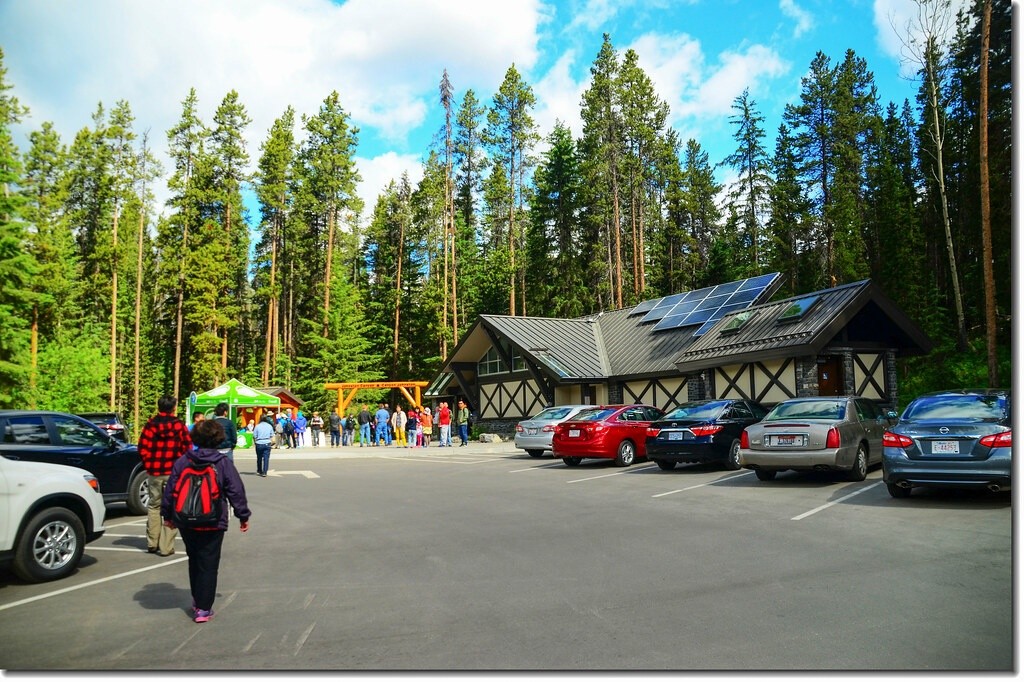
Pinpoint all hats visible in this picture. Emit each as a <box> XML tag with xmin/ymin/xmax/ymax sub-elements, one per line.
<box><xmin>313</xmin><ymin>412</ymin><xmax>317</xmax><ymax>416</ymax></box>
<box><xmin>362</xmin><ymin>405</ymin><xmax>367</xmax><ymax>409</ymax></box>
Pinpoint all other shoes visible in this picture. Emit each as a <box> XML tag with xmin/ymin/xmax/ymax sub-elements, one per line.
<box><xmin>162</xmin><ymin>547</ymin><xmax>175</xmax><ymax>557</ymax></box>
<box><xmin>257</xmin><ymin>472</ymin><xmax>266</xmax><ymax>477</ymax></box>
<box><xmin>275</xmin><ymin>442</ymin><xmax>467</xmax><ymax>449</ymax></box>
<box><xmin>148</xmin><ymin>546</ymin><xmax>161</xmax><ymax>552</ymax></box>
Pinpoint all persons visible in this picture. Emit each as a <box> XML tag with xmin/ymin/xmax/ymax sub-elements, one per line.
<box><xmin>341</xmin><ymin>414</ymin><xmax>357</xmax><ymax>446</ymax></box>
<box><xmin>137</xmin><ymin>395</ymin><xmax>192</xmax><ymax>555</ymax></box>
<box><xmin>391</xmin><ymin>404</ymin><xmax>408</xmax><ymax>448</ymax></box>
<box><xmin>246</xmin><ymin>420</ymin><xmax>255</xmax><ymax>431</ymax></box>
<box><xmin>435</xmin><ymin>402</ymin><xmax>454</xmax><ymax>447</ymax></box>
<box><xmin>266</xmin><ymin>411</ymin><xmax>275</xmax><ymax>425</ymax></box>
<box><xmin>406</xmin><ymin>406</ymin><xmax>433</xmax><ymax>448</ymax></box>
<box><xmin>162</xmin><ymin>420</ymin><xmax>250</xmax><ymax>622</ymax></box>
<box><xmin>276</xmin><ymin>409</ymin><xmax>307</xmax><ymax>449</ymax></box>
<box><xmin>458</xmin><ymin>401</ymin><xmax>469</xmax><ymax>446</ymax></box>
<box><xmin>253</xmin><ymin>417</ymin><xmax>275</xmax><ymax>476</ymax></box>
<box><xmin>310</xmin><ymin>412</ymin><xmax>324</xmax><ymax>447</ymax></box>
<box><xmin>358</xmin><ymin>404</ymin><xmax>393</xmax><ymax>447</ymax></box>
<box><xmin>329</xmin><ymin>410</ymin><xmax>341</xmax><ymax>447</ymax></box>
<box><xmin>188</xmin><ymin>412</ymin><xmax>204</xmax><ymax>450</ymax></box>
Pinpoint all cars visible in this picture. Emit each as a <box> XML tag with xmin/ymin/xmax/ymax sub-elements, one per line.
<box><xmin>0</xmin><ymin>455</ymin><xmax>110</xmax><ymax>585</ymax></box>
<box><xmin>738</xmin><ymin>395</ymin><xmax>899</xmax><ymax>482</ymax></box>
<box><xmin>512</xmin><ymin>404</ymin><xmax>600</xmax><ymax>458</ymax></box>
<box><xmin>645</xmin><ymin>398</ymin><xmax>770</xmax><ymax>471</ymax></box>
<box><xmin>552</xmin><ymin>404</ymin><xmax>667</xmax><ymax>467</ymax></box>
<box><xmin>66</xmin><ymin>411</ymin><xmax>131</xmax><ymax>444</ymax></box>
<box><xmin>0</xmin><ymin>408</ymin><xmax>152</xmax><ymax>516</ymax></box>
<box><xmin>881</xmin><ymin>387</ymin><xmax>1012</xmax><ymax>499</ymax></box>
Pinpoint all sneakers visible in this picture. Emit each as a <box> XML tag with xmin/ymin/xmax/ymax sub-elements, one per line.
<box><xmin>195</xmin><ymin>609</ymin><xmax>214</xmax><ymax>622</ymax></box>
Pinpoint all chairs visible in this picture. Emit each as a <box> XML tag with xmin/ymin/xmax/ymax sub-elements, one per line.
<box><xmin>627</xmin><ymin>412</ymin><xmax>636</xmax><ymax>420</ymax></box>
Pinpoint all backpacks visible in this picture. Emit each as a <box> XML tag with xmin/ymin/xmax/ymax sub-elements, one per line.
<box><xmin>463</xmin><ymin>408</ymin><xmax>474</xmax><ymax>426</ymax></box>
<box><xmin>276</xmin><ymin>421</ymin><xmax>283</xmax><ymax>433</ymax></box>
<box><xmin>173</xmin><ymin>456</ymin><xmax>232</xmax><ymax>529</ymax></box>
<box><xmin>346</xmin><ymin>418</ymin><xmax>355</xmax><ymax>429</ymax></box>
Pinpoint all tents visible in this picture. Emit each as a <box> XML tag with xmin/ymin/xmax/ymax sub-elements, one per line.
<box><xmin>185</xmin><ymin>378</ymin><xmax>281</xmax><ymax>450</ymax></box>
